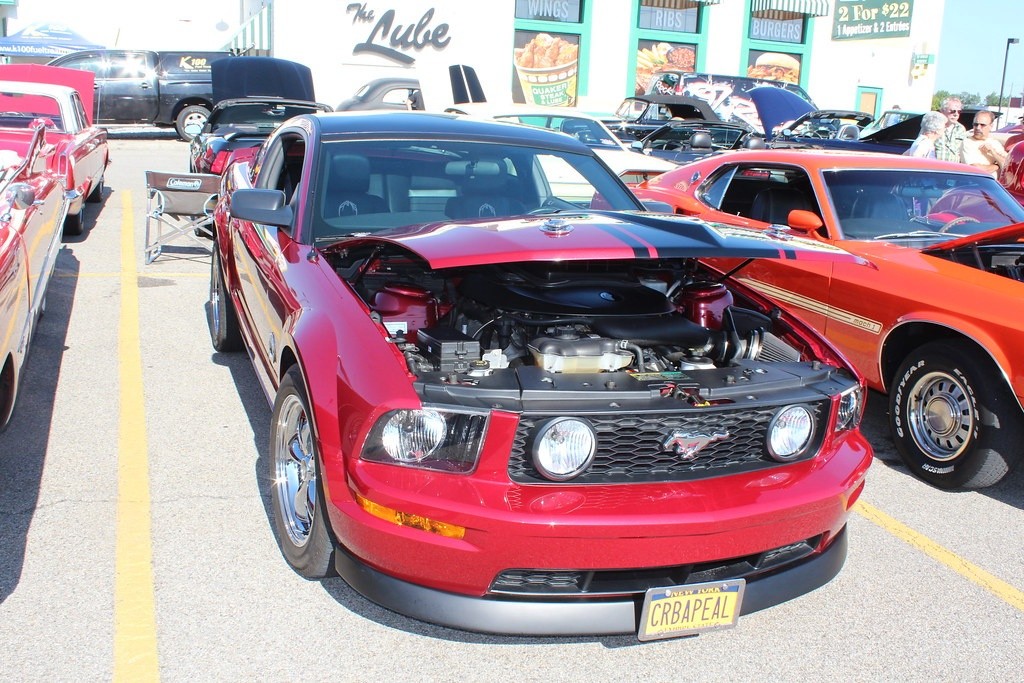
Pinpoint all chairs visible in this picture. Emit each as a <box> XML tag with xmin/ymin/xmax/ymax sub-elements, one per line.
<box><xmin>686</xmin><ymin>132</ymin><xmax>713</xmax><ymax>154</ymax></box>
<box><xmin>446</xmin><ymin>173</ymin><xmax>530</xmax><ymax>220</ymax></box>
<box><xmin>851</xmin><ymin>192</ymin><xmax>909</xmax><ymax>220</ymax></box>
<box><xmin>323</xmin><ymin>155</ymin><xmax>391</xmax><ymax>217</ymax></box>
<box><xmin>745</xmin><ymin>137</ymin><xmax>767</xmax><ymax>148</ymax></box>
<box><xmin>146</xmin><ymin>171</ymin><xmax>222</xmax><ymax>264</ymax></box>
<box><xmin>753</xmin><ymin>186</ymin><xmax>811</xmax><ymax>226</ymax></box>
<box><xmin>835</xmin><ymin>125</ymin><xmax>861</xmax><ymax>141</ymax></box>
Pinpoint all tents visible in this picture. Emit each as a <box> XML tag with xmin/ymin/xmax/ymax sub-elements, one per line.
<box><xmin>0</xmin><ymin>22</ymin><xmax>106</xmax><ymax>57</ymax></box>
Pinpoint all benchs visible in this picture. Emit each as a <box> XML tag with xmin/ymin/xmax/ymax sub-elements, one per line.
<box><xmin>288</xmin><ymin>155</ymin><xmax>470</xmax><ymax>212</ymax></box>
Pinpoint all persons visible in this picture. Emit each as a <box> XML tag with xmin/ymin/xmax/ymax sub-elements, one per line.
<box><xmin>901</xmin><ymin>96</ymin><xmax>1009</xmax><ymax>181</ymax></box>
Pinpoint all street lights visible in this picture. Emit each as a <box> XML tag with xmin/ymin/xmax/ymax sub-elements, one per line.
<box><xmin>995</xmin><ymin>37</ymin><xmax>1021</xmax><ymax>131</ymax></box>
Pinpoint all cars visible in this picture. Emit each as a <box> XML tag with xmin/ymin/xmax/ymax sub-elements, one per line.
<box><xmin>587</xmin><ymin>144</ymin><xmax>1024</xmax><ymax>496</ymax></box>
<box><xmin>0</xmin><ymin>116</ymin><xmax>71</xmax><ymax>432</ymax></box>
<box><xmin>208</xmin><ymin>108</ymin><xmax>877</xmax><ymax>642</ymax></box>
<box><xmin>0</xmin><ymin>64</ymin><xmax>112</xmax><ymax>236</ymax></box>
<box><xmin>188</xmin><ymin>56</ymin><xmax>336</xmax><ymax>237</ymax></box>
<box><xmin>340</xmin><ymin>62</ymin><xmax>1024</xmax><ymax>210</ymax></box>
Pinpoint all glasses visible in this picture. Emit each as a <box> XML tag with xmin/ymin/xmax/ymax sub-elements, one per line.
<box><xmin>944</xmin><ymin>109</ymin><xmax>962</xmax><ymax>114</ymax></box>
<box><xmin>972</xmin><ymin>123</ymin><xmax>990</xmax><ymax>127</ymax></box>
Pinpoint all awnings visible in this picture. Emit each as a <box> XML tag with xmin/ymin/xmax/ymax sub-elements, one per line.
<box><xmin>750</xmin><ymin>0</ymin><xmax>830</xmax><ymax>16</ymax></box>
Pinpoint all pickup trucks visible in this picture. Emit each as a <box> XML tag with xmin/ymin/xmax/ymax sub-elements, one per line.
<box><xmin>44</xmin><ymin>47</ymin><xmax>238</xmax><ymax>143</ymax></box>
